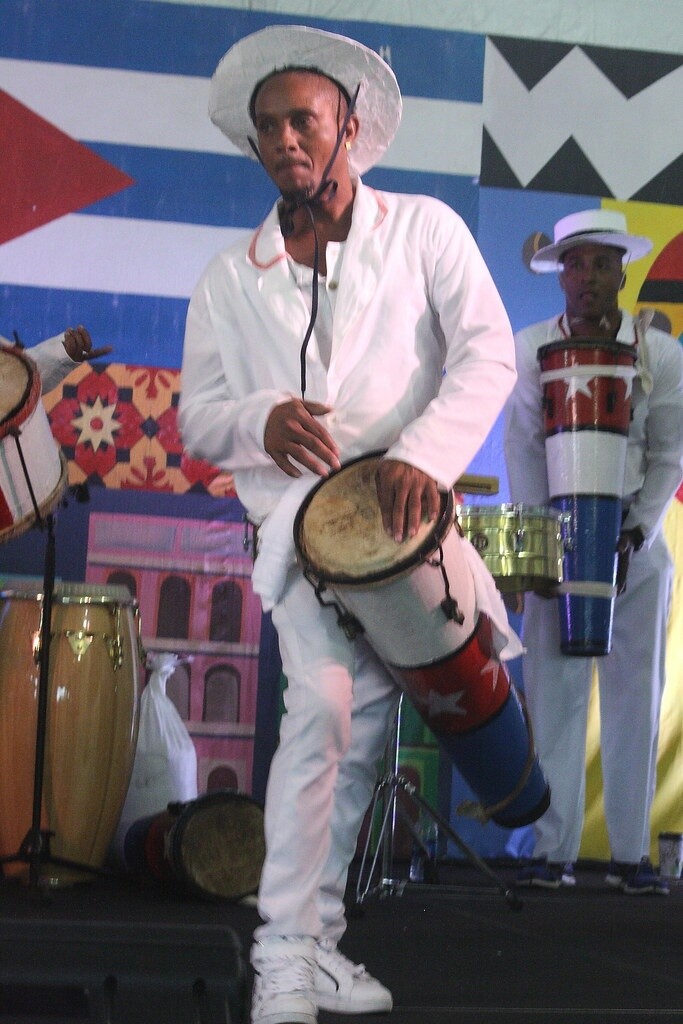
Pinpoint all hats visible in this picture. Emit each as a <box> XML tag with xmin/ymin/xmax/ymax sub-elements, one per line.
<box><xmin>209</xmin><ymin>24</ymin><xmax>402</xmax><ymax>175</ymax></box>
<box><xmin>530</xmin><ymin>209</ymin><xmax>651</xmax><ymax>272</ymax></box>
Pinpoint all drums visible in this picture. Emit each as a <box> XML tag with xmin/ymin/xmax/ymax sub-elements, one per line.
<box><xmin>0</xmin><ymin>332</ymin><xmax>71</xmax><ymax>544</ymax></box>
<box><xmin>0</xmin><ymin>575</ymin><xmax>144</xmax><ymax>891</ymax></box>
<box><xmin>457</xmin><ymin>500</ymin><xmax>570</xmax><ymax>595</ymax></box>
<box><xmin>538</xmin><ymin>340</ymin><xmax>639</xmax><ymax>660</ymax></box>
<box><xmin>294</xmin><ymin>446</ymin><xmax>552</xmax><ymax>827</ymax></box>
<box><xmin>125</xmin><ymin>789</ymin><xmax>270</xmax><ymax>902</ymax></box>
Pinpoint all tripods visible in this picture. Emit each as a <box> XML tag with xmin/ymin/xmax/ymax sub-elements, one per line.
<box><xmin>351</xmin><ymin>693</ymin><xmax>525</xmax><ymax>920</ymax></box>
<box><xmin>0</xmin><ymin>510</ymin><xmax>123</xmax><ymax>903</ymax></box>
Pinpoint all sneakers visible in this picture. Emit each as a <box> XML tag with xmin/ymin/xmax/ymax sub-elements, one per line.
<box><xmin>249</xmin><ymin>936</ymin><xmax>318</xmax><ymax>1024</ymax></box>
<box><xmin>603</xmin><ymin>855</ymin><xmax>671</xmax><ymax>894</ymax></box>
<box><xmin>313</xmin><ymin>948</ymin><xmax>393</xmax><ymax>1013</ymax></box>
<box><xmin>512</xmin><ymin>859</ymin><xmax>576</xmax><ymax>888</ymax></box>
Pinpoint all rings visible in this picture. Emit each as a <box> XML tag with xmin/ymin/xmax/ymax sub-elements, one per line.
<box><xmin>82</xmin><ymin>350</ymin><xmax>88</xmax><ymax>357</ymax></box>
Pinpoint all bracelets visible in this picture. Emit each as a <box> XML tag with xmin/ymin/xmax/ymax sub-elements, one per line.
<box><xmin>623</xmin><ymin>509</ymin><xmax>643</xmax><ymax>553</ymax></box>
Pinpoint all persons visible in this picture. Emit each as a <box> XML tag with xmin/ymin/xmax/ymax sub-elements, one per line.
<box><xmin>179</xmin><ymin>26</ymin><xmax>518</xmax><ymax>1024</ymax></box>
<box><xmin>0</xmin><ymin>326</ymin><xmax>111</xmax><ymax>395</ymax></box>
<box><xmin>505</xmin><ymin>211</ymin><xmax>683</xmax><ymax>895</ymax></box>
